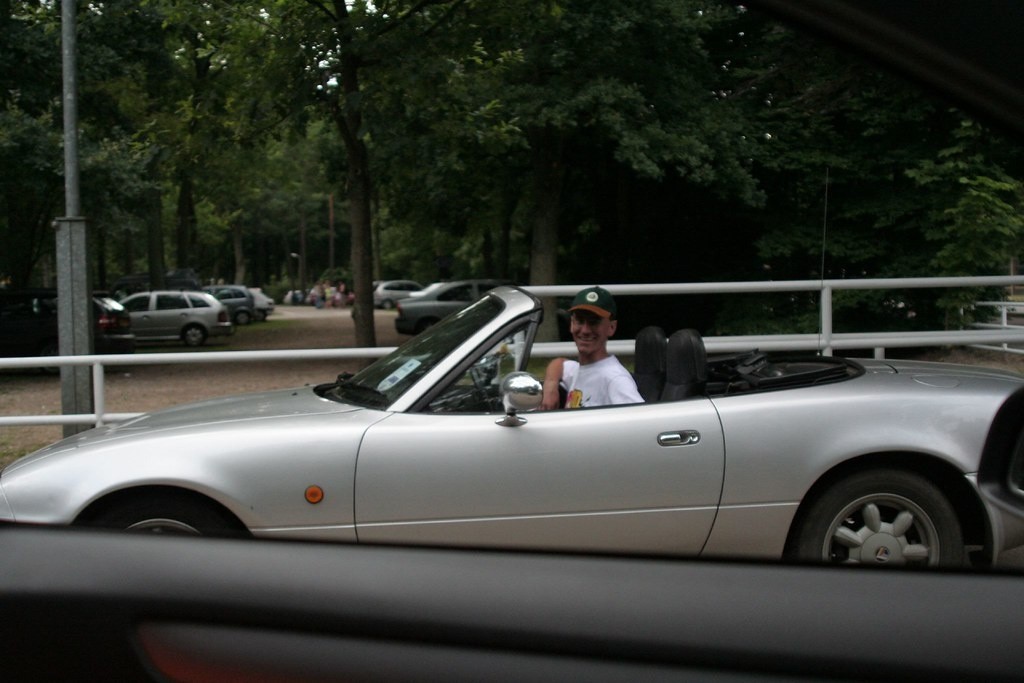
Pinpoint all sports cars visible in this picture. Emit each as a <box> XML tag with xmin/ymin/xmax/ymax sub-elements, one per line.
<box><xmin>0</xmin><ymin>278</ymin><xmax>1024</xmax><ymax>569</ymax></box>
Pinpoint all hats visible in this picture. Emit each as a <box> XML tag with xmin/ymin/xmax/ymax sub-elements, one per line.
<box><xmin>567</xmin><ymin>286</ymin><xmax>617</xmax><ymax>321</ymax></box>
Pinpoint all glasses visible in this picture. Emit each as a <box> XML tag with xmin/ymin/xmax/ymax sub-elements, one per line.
<box><xmin>571</xmin><ymin>315</ymin><xmax>602</xmax><ymax>324</ymax></box>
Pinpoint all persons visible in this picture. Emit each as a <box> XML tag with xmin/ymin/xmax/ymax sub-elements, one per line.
<box><xmin>534</xmin><ymin>285</ymin><xmax>646</xmax><ymax>411</ymax></box>
<box><xmin>285</xmin><ymin>279</ymin><xmax>348</xmax><ymax>308</ymax></box>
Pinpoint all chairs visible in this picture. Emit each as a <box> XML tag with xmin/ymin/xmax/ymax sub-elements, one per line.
<box><xmin>633</xmin><ymin>325</ymin><xmax>666</xmax><ymax>404</ymax></box>
<box><xmin>660</xmin><ymin>329</ymin><xmax>707</xmax><ymax>403</ymax></box>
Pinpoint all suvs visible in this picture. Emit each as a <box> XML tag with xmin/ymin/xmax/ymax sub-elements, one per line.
<box><xmin>116</xmin><ymin>291</ymin><xmax>234</xmax><ymax>347</ymax></box>
<box><xmin>1</xmin><ymin>283</ymin><xmax>136</xmax><ymax>372</ymax></box>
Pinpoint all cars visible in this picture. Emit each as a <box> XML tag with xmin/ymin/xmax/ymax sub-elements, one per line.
<box><xmin>395</xmin><ymin>279</ymin><xmax>505</xmax><ymax>337</ymax></box>
<box><xmin>372</xmin><ymin>280</ymin><xmax>427</xmax><ymax>310</ymax></box>
<box><xmin>206</xmin><ymin>284</ymin><xmax>275</xmax><ymax>324</ymax></box>
